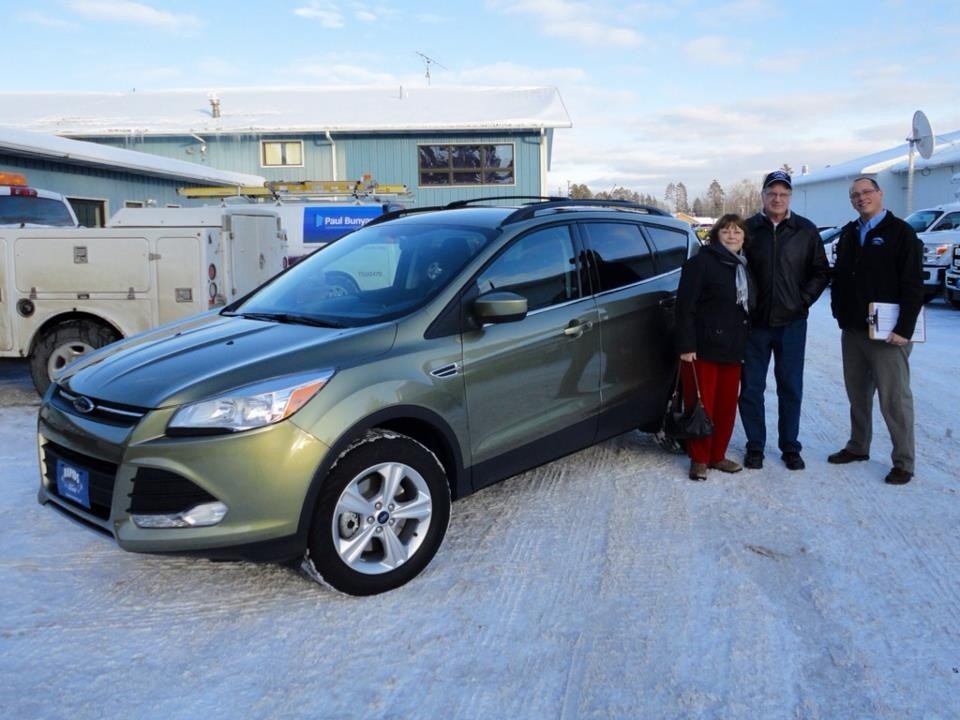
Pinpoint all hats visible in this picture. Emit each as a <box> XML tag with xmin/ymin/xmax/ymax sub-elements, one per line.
<box><xmin>764</xmin><ymin>171</ymin><xmax>793</xmax><ymax>191</ymax></box>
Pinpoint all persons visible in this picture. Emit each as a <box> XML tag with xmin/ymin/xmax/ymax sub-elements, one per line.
<box><xmin>676</xmin><ymin>215</ymin><xmax>751</xmax><ymax>481</ymax></box>
<box><xmin>569</xmin><ymin>249</ymin><xmax>642</xmax><ymax>296</ymax></box>
<box><xmin>738</xmin><ymin>170</ymin><xmax>831</xmax><ymax>469</ymax></box>
<box><xmin>828</xmin><ymin>177</ymin><xmax>924</xmax><ymax>483</ymax></box>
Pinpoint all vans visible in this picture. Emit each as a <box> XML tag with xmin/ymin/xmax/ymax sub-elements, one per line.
<box><xmin>0</xmin><ymin>173</ymin><xmax>87</xmax><ymax>230</ymax></box>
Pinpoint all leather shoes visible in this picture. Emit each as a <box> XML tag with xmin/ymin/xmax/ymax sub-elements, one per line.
<box><xmin>689</xmin><ymin>462</ymin><xmax>708</xmax><ymax>480</ymax></box>
<box><xmin>885</xmin><ymin>467</ymin><xmax>913</xmax><ymax>484</ymax></box>
<box><xmin>781</xmin><ymin>452</ymin><xmax>805</xmax><ymax>470</ymax></box>
<box><xmin>829</xmin><ymin>448</ymin><xmax>870</xmax><ymax>463</ymax></box>
<box><xmin>708</xmin><ymin>458</ymin><xmax>743</xmax><ymax>473</ymax></box>
<box><xmin>744</xmin><ymin>450</ymin><xmax>765</xmax><ymax>468</ymax></box>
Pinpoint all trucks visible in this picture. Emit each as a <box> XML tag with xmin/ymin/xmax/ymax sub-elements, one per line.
<box><xmin>901</xmin><ymin>206</ymin><xmax>960</xmax><ymax>310</ymax></box>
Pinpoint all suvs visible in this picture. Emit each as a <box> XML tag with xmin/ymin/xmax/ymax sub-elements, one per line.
<box><xmin>38</xmin><ymin>196</ymin><xmax>705</xmax><ymax>597</ymax></box>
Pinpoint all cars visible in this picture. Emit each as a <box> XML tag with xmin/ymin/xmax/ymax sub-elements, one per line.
<box><xmin>820</xmin><ymin>227</ymin><xmax>841</xmax><ymax>268</ymax></box>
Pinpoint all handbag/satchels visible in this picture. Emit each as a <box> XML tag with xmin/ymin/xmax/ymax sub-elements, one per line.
<box><xmin>661</xmin><ymin>359</ymin><xmax>714</xmax><ymax>447</ymax></box>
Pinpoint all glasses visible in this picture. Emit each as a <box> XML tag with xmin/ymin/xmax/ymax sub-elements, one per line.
<box><xmin>849</xmin><ymin>188</ymin><xmax>879</xmax><ymax>200</ymax></box>
